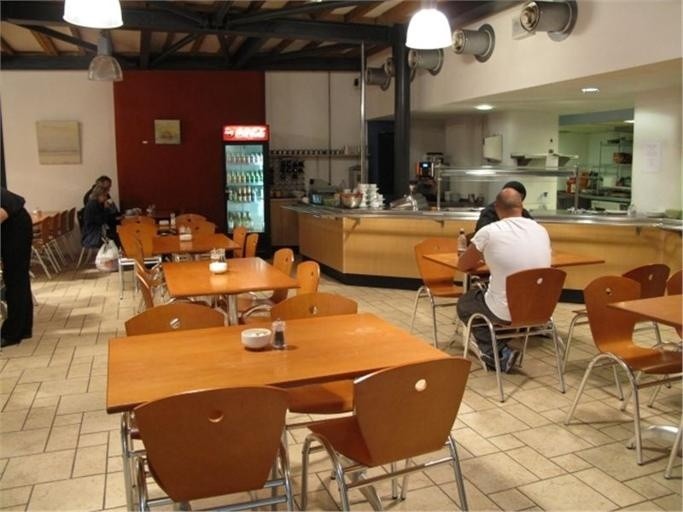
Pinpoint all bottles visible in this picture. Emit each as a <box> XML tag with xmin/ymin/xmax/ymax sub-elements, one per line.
<box><xmin>456</xmin><ymin>229</ymin><xmax>467</xmax><ymax>261</ymax></box>
<box><xmin>271</xmin><ymin>317</ymin><xmax>287</xmax><ymax>349</ymax></box>
<box><xmin>159</xmin><ymin>211</ymin><xmax>192</xmax><ymax>237</ymax></box>
<box><xmin>224</xmin><ymin>148</ymin><xmax>263</xmax><ymax>232</ymax></box>
<box><xmin>208</xmin><ymin>247</ymin><xmax>224</xmax><ymax>262</ymax></box>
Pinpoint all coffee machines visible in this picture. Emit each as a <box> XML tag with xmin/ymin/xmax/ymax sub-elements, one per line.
<box><xmin>415</xmin><ymin>151</ymin><xmax>450</xmax><ymax>201</ymax></box>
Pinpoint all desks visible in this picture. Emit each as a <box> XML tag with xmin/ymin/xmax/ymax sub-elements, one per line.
<box><xmin>605</xmin><ymin>293</ymin><xmax>681</xmax><ymax>340</ymax></box>
<box><xmin>422</xmin><ymin>249</ymin><xmax>604</xmax><ymax>275</ymax></box>
<box><xmin>107</xmin><ymin>311</ymin><xmax>456</xmax><ymax>415</ymax></box>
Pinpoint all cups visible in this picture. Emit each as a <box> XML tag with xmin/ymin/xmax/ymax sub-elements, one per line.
<box><xmin>269</xmin><ymin>189</ymin><xmax>287</xmax><ymax>198</ymax></box>
<box><xmin>467</xmin><ymin>193</ymin><xmax>475</xmax><ymax>204</ymax></box>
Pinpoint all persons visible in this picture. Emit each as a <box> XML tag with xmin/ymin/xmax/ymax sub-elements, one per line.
<box><xmin>0</xmin><ymin>187</ymin><xmax>33</xmax><ymax>346</ymax></box>
<box><xmin>457</xmin><ymin>187</ymin><xmax>551</xmax><ymax>373</ymax></box>
<box><xmin>80</xmin><ymin>186</ymin><xmax>123</xmax><ymax>248</ymax></box>
<box><xmin>475</xmin><ymin>181</ymin><xmax>535</xmax><ymax>238</ymax></box>
<box><xmin>83</xmin><ymin>175</ymin><xmax>118</xmax><ymax>215</ymax></box>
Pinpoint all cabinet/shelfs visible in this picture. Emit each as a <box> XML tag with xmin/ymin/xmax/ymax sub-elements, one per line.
<box><xmin>596</xmin><ymin>138</ymin><xmax>631</xmax><ymax>196</ymax></box>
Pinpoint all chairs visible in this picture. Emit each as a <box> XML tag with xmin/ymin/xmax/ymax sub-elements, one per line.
<box><xmin>29</xmin><ymin>207</ymin><xmax>77</xmax><ymax>279</ymax></box>
<box><xmin>271</xmin><ymin>292</ymin><xmax>400</xmax><ymax>510</ymax></box>
<box><xmin>665</xmin><ymin>425</ymin><xmax>680</xmax><ymax>480</ymax></box>
<box><xmin>564</xmin><ymin>276</ymin><xmax>681</xmax><ymax>465</ymax></box>
<box><xmin>463</xmin><ymin>267</ymin><xmax>567</xmax><ymax>404</ymax></box>
<box><xmin>645</xmin><ymin>271</ymin><xmax>681</xmax><ymax>409</ymax></box>
<box><xmin>409</xmin><ymin>235</ymin><xmax>467</xmax><ymax>351</ymax></box>
<box><xmin>132</xmin><ymin>385</ymin><xmax>293</xmax><ymax>511</ymax></box>
<box><xmin>560</xmin><ymin>264</ymin><xmax>671</xmax><ymax>375</ymax></box>
<box><xmin>120</xmin><ymin>301</ymin><xmax>229</xmax><ymax>487</ymax></box>
<box><xmin>300</xmin><ymin>358</ymin><xmax>471</xmax><ymax>512</ymax></box>
<box><xmin>115</xmin><ymin>207</ymin><xmax>322</xmax><ymax>325</ymax></box>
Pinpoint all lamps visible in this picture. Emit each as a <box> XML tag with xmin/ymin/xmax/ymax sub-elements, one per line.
<box><xmin>59</xmin><ymin>0</ymin><xmax>124</xmax><ymax>30</ymax></box>
<box><xmin>83</xmin><ymin>37</ymin><xmax>124</xmax><ymax>85</ymax></box>
<box><xmin>403</xmin><ymin>0</ymin><xmax>456</xmax><ymax>53</ymax></box>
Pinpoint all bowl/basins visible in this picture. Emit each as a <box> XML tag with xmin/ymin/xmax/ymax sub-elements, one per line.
<box><xmin>442</xmin><ymin>191</ymin><xmax>460</xmax><ymax>203</ymax></box>
<box><xmin>208</xmin><ymin>261</ymin><xmax>227</xmax><ymax>275</ymax></box>
<box><xmin>292</xmin><ymin>183</ymin><xmax>385</xmax><ymax>209</ymax></box>
<box><xmin>241</xmin><ymin>328</ymin><xmax>272</xmax><ymax>350</ymax></box>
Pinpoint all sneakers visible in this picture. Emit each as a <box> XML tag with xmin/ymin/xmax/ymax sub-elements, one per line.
<box><xmin>481</xmin><ymin>349</ymin><xmax>499</xmax><ymax>368</ymax></box>
<box><xmin>499</xmin><ymin>346</ymin><xmax>519</xmax><ymax>374</ymax></box>
